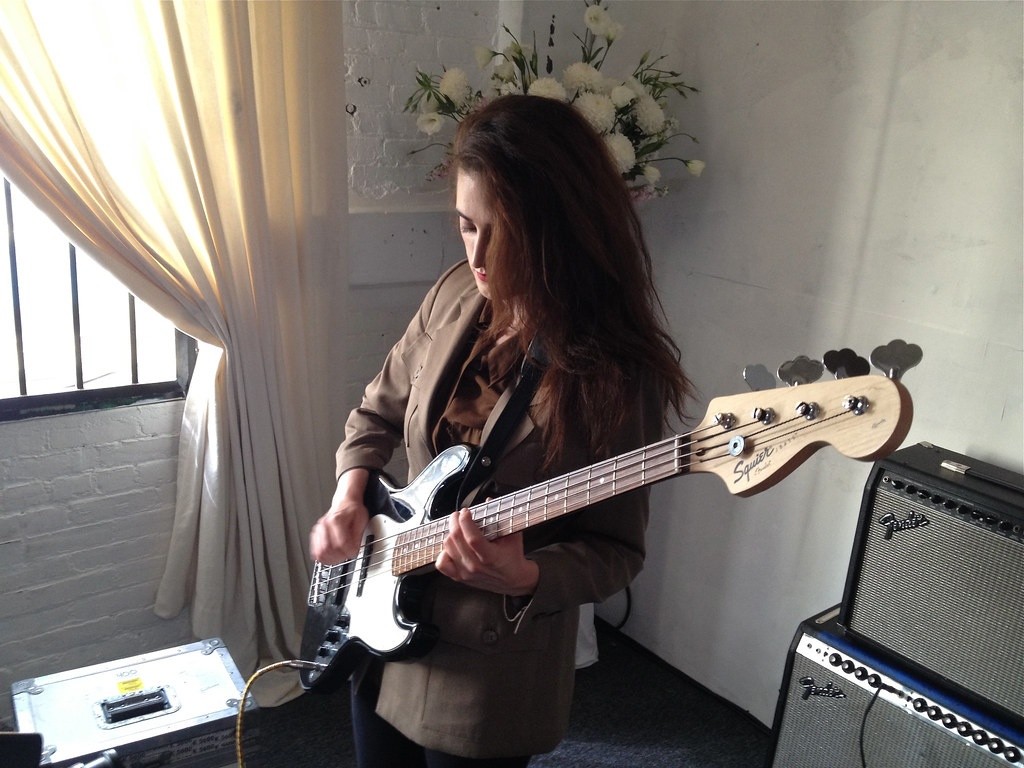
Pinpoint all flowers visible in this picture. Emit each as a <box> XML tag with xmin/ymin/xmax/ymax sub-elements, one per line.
<box><xmin>404</xmin><ymin>0</ymin><xmax>705</xmax><ymax>208</ymax></box>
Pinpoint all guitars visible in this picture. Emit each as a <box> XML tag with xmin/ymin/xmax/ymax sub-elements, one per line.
<box><xmin>295</xmin><ymin>337</ymin><xmax>926</xmax><ymax>700</ymax></box>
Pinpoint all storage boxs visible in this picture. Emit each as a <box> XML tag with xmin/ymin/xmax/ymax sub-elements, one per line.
<box><xmin>9</xmin><ymin>637</ymin><xmax>258</xmax><ymax>768</ymax></box>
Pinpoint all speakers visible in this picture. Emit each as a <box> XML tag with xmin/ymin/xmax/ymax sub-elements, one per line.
<box><xmin>837</xmin><ymin>441</ymin><xmax>1024</xmax><ymax>733</ymax></box>
<box><xmin>764</xmin><ymin>602</ymin><xmax>1024</xmax><ymax>768</ymax></box>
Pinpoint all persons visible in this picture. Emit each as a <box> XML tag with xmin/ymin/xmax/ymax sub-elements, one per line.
<box><xmin>310</xmin><ymin>94</ymin><xmax>699</xmax><ymax>768</ymax></box>
